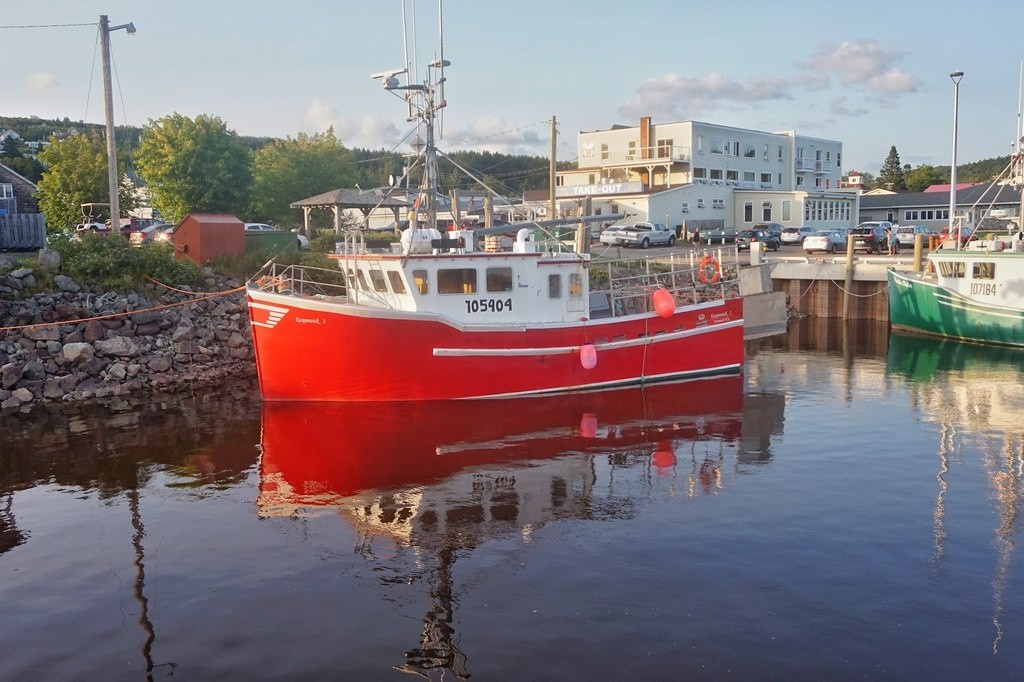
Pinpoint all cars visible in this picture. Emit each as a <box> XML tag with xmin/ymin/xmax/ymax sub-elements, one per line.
<box><xmin>894</xmin><ymin>224</ymin><xmax>937</xmax><ymax>249</ymax></box>
<box><xmin>129</xmin><ymin>224</ymin><xmax>175</xmax><ymax>249</ymax></box>
<box><xmin>735</xmin><ymin>228</ymin><xmax>780</xmax><ymax>252</ymax></box>
<box><xmin>780</xmin><ymin>226</ymin><xmax>815</xmax><ymax>246</ymax></box>
<box><xmin>244</xmin><ymin>222</ymin><xmax>309</xmax><ymax>248</ymax></box>
<box><xmin>154</xmin><ymin>226</ymin><xmax>175</xmax><ymax>243</ymax></box>
<box><xmin>937</xmin><ymin>227</ymin><xmax>978</xmax><ymax>248</ymax></box>
<box><xmin>599</xmin><ymin>225</ymin><xmax>633</xmax><ymax>246</ymax></box>
<box><xmin>803</xmin><ymin>229</ymin><xmax>847</xmax><ymax>255</ymax></box>
<box><xmin>847</xmin><ymin>226</ymin><xmax>901</xmax><ymax>257</ymax></box>
<box><xmin>77</xmin><ymin>216</ymin><xmax>169</xmax><ymax>242</ymax></box>
<box><xmin>382</xmin><ymin>218</ymin><xmax>532</xmax><ymax>241</ymax></box>
<box><xmin>833</xmin><ymin>227</ymin><xmax>854</xmax><ymax>237</ymax></box>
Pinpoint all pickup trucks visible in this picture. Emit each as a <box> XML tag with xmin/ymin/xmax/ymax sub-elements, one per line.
<box><xmin>617</xmin><ymin>221</ymin><xmax>677</xmax><ymax>249</ymax></box>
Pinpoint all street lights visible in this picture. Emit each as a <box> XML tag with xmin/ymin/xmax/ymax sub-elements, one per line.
<box><xmin>99</xmin><ymin>14</ymin><xmax>137</xmax><ymax>235</ymax></box>
<box><xmin>949</xmin><ymin>70</ymin><xmax>964</xmax><ymax>241</ymax></box>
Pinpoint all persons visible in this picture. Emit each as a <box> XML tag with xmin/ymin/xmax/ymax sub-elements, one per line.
<box><xmin>693</xmin><ymin>227</ymin><xmax>701</xmax><ymax>254</ymax></box>
<box><xmin>887</xmin><ymin>227</ymin><xmax>896</xmax><ymax>256</ymax></box>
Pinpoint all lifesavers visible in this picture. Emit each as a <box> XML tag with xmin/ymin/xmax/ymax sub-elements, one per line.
<box><xmin>698</xmin><ymin>257</ymin><xmax>720</xmax><ymax>284</ymax></box>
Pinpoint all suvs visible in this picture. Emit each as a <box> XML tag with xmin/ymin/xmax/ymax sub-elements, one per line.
<box><xmin>858</xmin><ymin>220</ymin><xmax>893</xmax><ymax>234</ymax></box>
<box><xmin>753</xmin><ymin>222</ymin><xmax>786</xmax><ymax>240</ymax></box>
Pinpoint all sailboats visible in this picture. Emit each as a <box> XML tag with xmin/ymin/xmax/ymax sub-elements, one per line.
<box><xmin>885</xmin><ymin>58</ymin><xmax>1024</xmax><ymax>351</ymax></box>
<box><xmin>884</xmin><ymin>326</ymin><xmax>1024</xmax><ymax>656</ymax></box>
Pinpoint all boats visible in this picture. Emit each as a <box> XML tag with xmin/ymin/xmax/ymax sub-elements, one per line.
<box><xmin>245</xmin><ymin>0</ymin><xmax>747</xmax><ymax>404</ymax></box>
<box><xmin>251</xmin><ymin>364</ymin><xmax>746</xmax><ymax>682</ymax></box>
